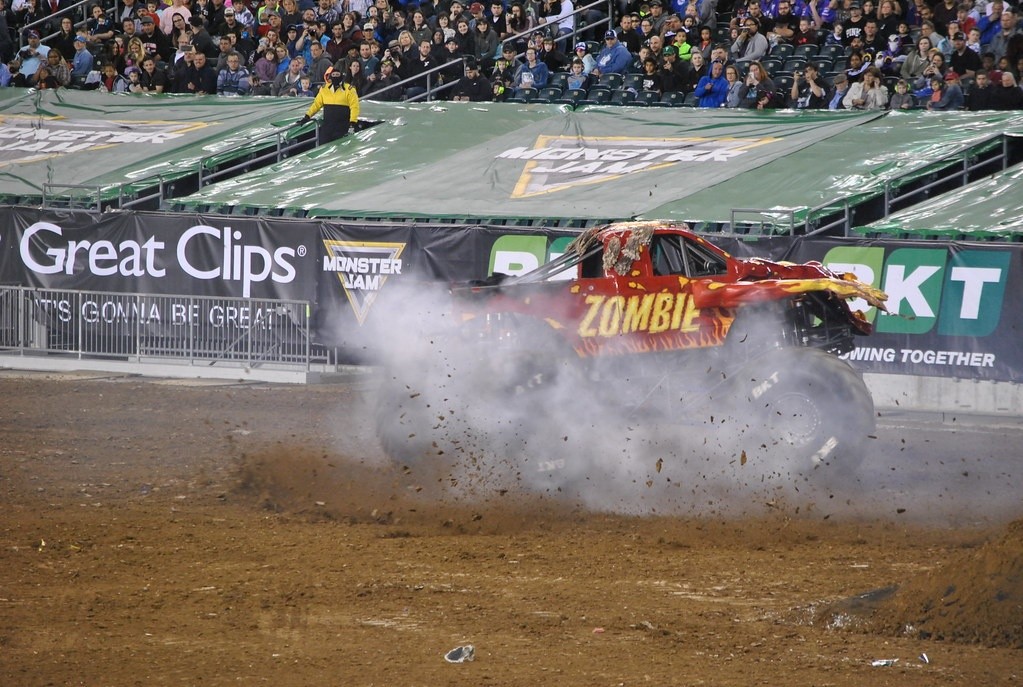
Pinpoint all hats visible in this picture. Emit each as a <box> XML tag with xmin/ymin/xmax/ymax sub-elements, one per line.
<box><xmin>446</xmin><ymin>36</ymin><xmax>458</xmax><ymax>45</ymax></box>
<box><xmin>649</xmin><ymin>0</ymin><xmax>662</xmax><ymax>8</ymax></box>
<box><xmin>850</xmin><ymin>1</ymin><xmax>860</xmax><ymax>9</ymax></box>
<box><xmin>574</xmin><ymin>42</ymin><xmax>588</xmax><ymax>51</ymax></box>
<box><xmin>630</xmin><ymin>12</ymin><xmax>641</xmax><ymax>17</ymax></box>
<box><xmin>605</xmin><ymin>29</ymin><xmax>617</xmax><ymax>39</ymax></box>
<box><xmin>671</xmin><ymin>12</ymin><xmax>683</xmax><ymax>22</ymax></box>
<box><xmin>136</xmin><ymin>3</ymin><xmax>148</xmax><ymax>11</ymax></box>
<box><xmin>389</xmin><ymin>40</ymin><xmax>401</xmax><ymax>49</ymax></box>
<box><xmin>833</xmin><ymin>74</ymin><xmax>847</xmax><ymax>84</ymax></box>
<box><xmin>347</xmin><ymin>43</ymin><xmax>360</xmax><ymax>53</ymax></box>
<box><xmin>140</xmin><ymin>16</ymin><xmax>154</xmax><ymax>24</ymax></box>
<box><xmin>364</xmin><ymin>23</ymin><xmax>374</xmax><ymax>31</ymax></box>
<box><xmin>224</xmin><ymin>8</ymin><xmax>235</xmax><ymax>17</ymax></box>
<box><xmin>543</xmin><ymin>37</ymin><xmax>553</xmax><ymax>44</ymax></box>
<box><xmin>268</xmin><ymin>11</ymin><xmax>281</xmax><ymax>17</ymax></box>
<box><xmin>188</xmin><ymin>16</ymin><xmax>203</xmax><ymax>27</ymax></box>
<box><xmin>945</xmin><ymin>72</ymin><xmax>959</xmax><ymax>80</ymax></box>
<box><xmin>952</xmin><ymin>32</ymin><xmax>966</xmax><ymax>41</ymax></box>
<box><xmin>450</xmin><ymin>0</ymin><xmax>462</xmax><ymax>6</ymax></box>
<box><xmin>663</xmin><ymin>46</ymin><xmax>675</xmax><ymax>57</ymax></box>
<box><xmin>503</xmin><ymin>44</ymin><xmax>514</xmax><ymax>53</ymax></box>
<box><xmin>532</xmin><ymin>30</ymin><xmax>544</xmax><ymax>43</ymax></box>
<box><xmin>287</xmin><ymin>24</ymin><xmax>297</xmax><ymax>33</ymax></box>
<box><xmin>470</xmin><ymin>3</ymin><xmax>485</xmax><ymax>13</ymax></box>
<box><xmin>466</xmin><ymin>61</ymin><xmax>478</xmax><ymax>71</ymax></box>
<box><xmin>382</xmin><ymin>60</ymin><xmax>393</xmax><ymax>66</ymax></box>
<box><xmin>73</xmin><ymin>36</ymin><xmax>86</xmax><ymax>43</ymax></box>
<box><xmin>28</xmin><ymin>30</ymin><xmax>40</xmax><ymax>39</ymax></box>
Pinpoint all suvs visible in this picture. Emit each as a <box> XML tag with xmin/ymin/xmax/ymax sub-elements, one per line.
<box><xmin>375</xmin><ymin>221</ymin><xmax>889</xmax><ymax>498</ymax></box>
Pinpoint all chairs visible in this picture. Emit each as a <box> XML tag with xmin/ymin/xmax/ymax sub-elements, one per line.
<box><xmin>0</xmin><ymin>0</ymin><xmax>1023</xmax><ymax>240</ymax></box>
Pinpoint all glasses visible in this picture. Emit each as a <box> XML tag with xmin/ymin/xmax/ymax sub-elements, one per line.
<box><xmin>631</xmin><ymin>20</ymin><xmax>640</xmax><ymax>22</ymax></box>
<box><xmin>746</xmin><ymin>24</ymin><xmax>756</xmax><ymax>27</ymax></box>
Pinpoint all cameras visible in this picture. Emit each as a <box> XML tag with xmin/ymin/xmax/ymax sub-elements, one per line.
<box><xmin>389</xmin><ymin>49</ymin><xmax>399</xmax><ymax>58</ymax></box>
<box><xmin>308</xmin><ymin>29</ymin><xmax>316</xmax><ymax>36</ymax></box>
<box><xmin>19</xmin><ymin>50</ymin><xmax>32</xmax><ymax>59</ymax></box>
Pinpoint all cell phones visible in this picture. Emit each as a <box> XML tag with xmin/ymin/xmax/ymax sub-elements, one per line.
<box><xmin>180</xmin><ymin>46</ymin><xmax>192</xmax><ymax>50</ymax></box>
<box><xmin>748</xmin><ymin>72</ymin><xmax>753</xmax><ymax>77</ymax></box>
<box><xmin>796</xmin><ymin>70</ymin><xmax>807</xmax><ymax>77</ymax></box>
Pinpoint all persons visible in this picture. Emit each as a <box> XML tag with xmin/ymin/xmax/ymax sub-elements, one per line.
<box><xmin>0</xmin><ymin>0</ymin><xmax>1023</xmax><ymax>111</ymax></box>
<box><xmin>296</xmin><ymin>70</ymin><xmax>360</xmax><ymax>146</ymax></box>
